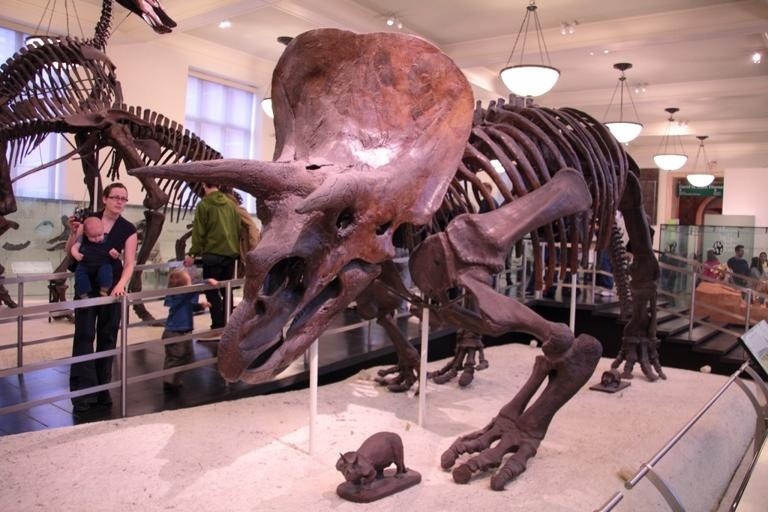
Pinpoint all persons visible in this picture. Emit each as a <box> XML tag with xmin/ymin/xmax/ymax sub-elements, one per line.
<box><xmin>161</xmin><ymin>269</ymin><xmax>218</xmax><ymax>392</ymax></box>
<box><xmin>65</xmin><ymin>183</ymin><xmax>138</xmax><ymax>412</ymax></box>
<box><xmin>479</xmin><ymin>182</ymin><xmax>767</xmax><ymax>304</ymax></box>
<box><xmin>70</xmin><ymin>215</ymin><xmax>120</xmax><ymax>300</ymax></box>
<box><xmin>184</xmin><ymin>181</ymin><xmax>260</xmax><ymax>330</ymax></box>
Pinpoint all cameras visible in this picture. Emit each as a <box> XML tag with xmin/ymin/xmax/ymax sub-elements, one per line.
<box><xmin>75</xmin><ymin>207</ymin><xmax>90</xmax><ymax>224</ymax></box>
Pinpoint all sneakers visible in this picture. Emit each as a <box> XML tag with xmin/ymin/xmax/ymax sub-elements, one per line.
<box><xmin>595</xmin><ymin>289</ymin><xmax>615</xmax><ymax>297</ymax></box>
<box><xmin>197</xmin><ymin>332</ymin><xmax>221</xmax><ymax>342</ymax></box>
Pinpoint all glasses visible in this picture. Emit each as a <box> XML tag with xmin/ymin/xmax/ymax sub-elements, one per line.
<box><xmin>107</xmin><ymin>195</ymin><xmax>129</xmax><ymax>201</ymax></box>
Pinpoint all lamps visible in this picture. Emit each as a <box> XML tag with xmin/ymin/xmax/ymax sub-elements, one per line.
<box><xmin>686</xmin><ymin>135</ymin><xmax>715</xmax><ymax>188</ymax></box>
<box><xmin>499</xmin><ymin>1</ymin><xmax>560</xmax><ymax>97</ymax></box>
<box><xmin>599</xmin><ymin>63</ymin><xmax>644</xmax><ymax>144</ymax></box>
<box><xmin>260</xmin><ymin>36</ymin><xmax>295</xmax><ymax>119</ymax></box>
<box><xmin>653</xmin><ymin>108</ymin><xmax>689</xmax><ymax>172</ymax></box>
<box><xmin>25</xmin><ymin>0</ymin><xmax>85</xmax><ymax>70</ymax></box>
